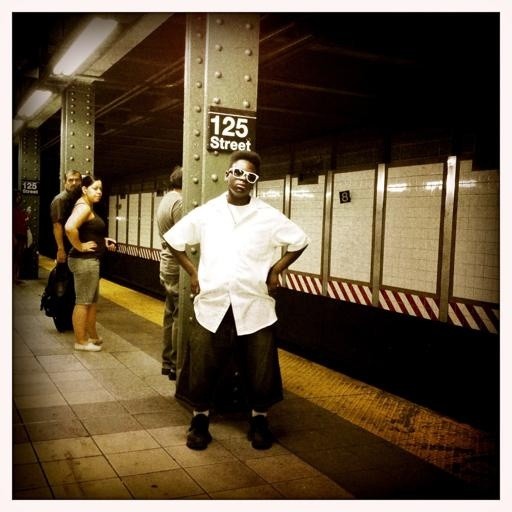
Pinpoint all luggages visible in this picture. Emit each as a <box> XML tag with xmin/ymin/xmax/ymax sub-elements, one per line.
<box><xmin>19</xmin><ymin>250</ymin><xmax>39</xmax><ymax>279</ymax></box>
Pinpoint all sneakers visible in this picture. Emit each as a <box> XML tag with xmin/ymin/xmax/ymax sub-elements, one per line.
<box><xmin>186</xmin><ymin>414</ymin><xmax>212</xmax><ymax>450</ymax></box>
<box><xmin>247</xmin><ymin>415</ymin><xmax>274</xmax><ymax>450</ymax></box>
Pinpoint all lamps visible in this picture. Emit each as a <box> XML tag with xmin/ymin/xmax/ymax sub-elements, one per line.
<box><xmin>13</xmin><ymin>11</ymin><xmax>118</xmax><ymax>132</ymax></box>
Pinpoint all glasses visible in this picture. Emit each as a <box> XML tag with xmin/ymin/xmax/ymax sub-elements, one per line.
<box><xmin>226</xmin><ymin>168</ymin><xmax>260</xmax><ymax>185</ymax></box>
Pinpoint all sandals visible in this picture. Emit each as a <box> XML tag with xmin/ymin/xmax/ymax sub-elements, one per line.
<box><xmin>73</xmin><ymin>335</ymin><xmax>105</xmax><ymax>352</ymax></box>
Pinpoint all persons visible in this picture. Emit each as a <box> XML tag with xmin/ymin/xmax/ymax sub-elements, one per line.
<box><xmin>50</xmin><ymin>169</ymin><xmax>116</xmax><ymax>353</ymax></box>
<box><xmin>163</xmin><ymin>152</ymin><xmax>310</xmax><ymax>450</ymax></box>
<box><xmin>154</xmin><ymin>164</ymin><xmax>183</xmax><ymax>380</ymax></box>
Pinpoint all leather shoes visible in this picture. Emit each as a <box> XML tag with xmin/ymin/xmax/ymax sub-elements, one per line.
<box><xmin>160</xmin><ymin>367</ymin><xmax>176</xmax><ymax>380</ymax></box>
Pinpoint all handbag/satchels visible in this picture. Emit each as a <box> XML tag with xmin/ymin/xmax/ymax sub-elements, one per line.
<box><xmin>40</xmin><ymin>264</ymin><xmax>66</xmax><ymax>319</ymax></box>
